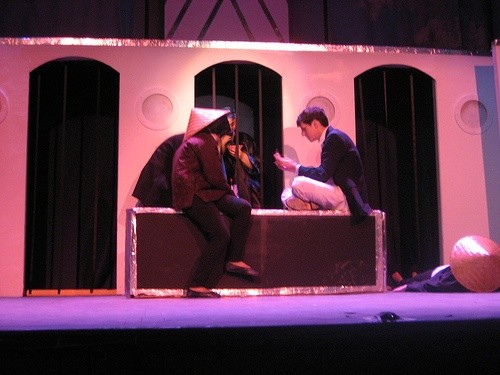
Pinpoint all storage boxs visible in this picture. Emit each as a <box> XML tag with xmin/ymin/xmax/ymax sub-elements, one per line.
<box><xmin>124</xmin><ymin>208</ymin><xmax>387</xmax><ymax>298</ymax></box>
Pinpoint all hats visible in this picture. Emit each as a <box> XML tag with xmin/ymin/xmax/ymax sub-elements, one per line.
<box><xmin>449</xmin><ymin>234</ymin><xmax>500</xmax><ymax>293</ymax></box>
<box><xmin>183</xmin><ymin>107</ymin><xmax>230</xmax><ymax>143</ymax></box>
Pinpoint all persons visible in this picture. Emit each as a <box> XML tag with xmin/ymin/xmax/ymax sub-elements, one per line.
<box><xmin>131</xmin><ymin>106</ymin><xmax>259</xmax><ymax>298</ymax></box>
<box><xmin>392</xmin><ymin>237</ymin><xmax>500</xmax><ymax>292</ymax></box>
<box><xmin>273</xmin><ymin>107</ymin><xmax>372</xmax><ymax>221</ymax></box>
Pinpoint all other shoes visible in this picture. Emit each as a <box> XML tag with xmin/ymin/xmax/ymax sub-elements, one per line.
<box><xmin>224</xmin><ymin>263</ymin><xmax>259</xmax><ymax>278</ymax></box>
<box><xmin>186</xmin><ymin>287</ymin><xmax>221</xmax><ymax>298</ymax></box>
<box><xmin>286</xmin><ymin>195</ymin><xmax>321</xmax><ymax>211</ymax></box>
